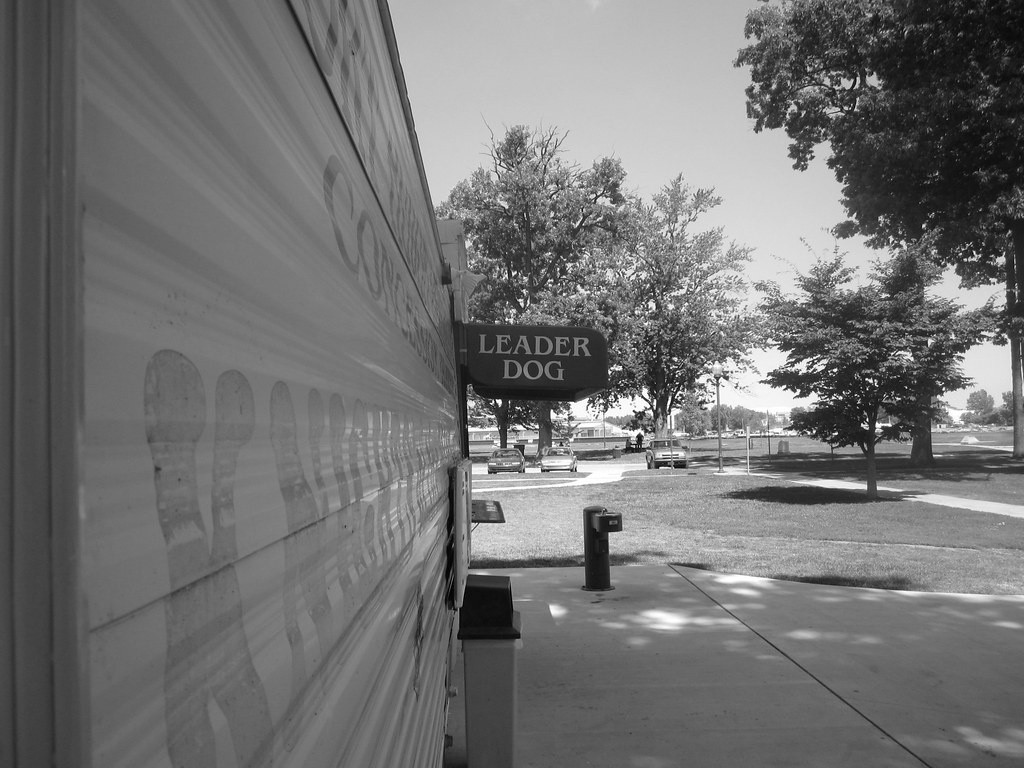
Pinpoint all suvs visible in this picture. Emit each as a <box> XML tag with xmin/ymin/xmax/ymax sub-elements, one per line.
<box><xmin>645</xmin><ymin>439</ymin><xmax>688</xmax><ymax>469</ymax></box>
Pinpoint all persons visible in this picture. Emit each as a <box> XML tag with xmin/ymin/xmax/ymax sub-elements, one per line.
<box><xmin>626</xmin><ymin>438</ymin><xmax>635</xmax><ymax>453</ymax></box>
<box><xmin>636</xmin><ymin>432</ymin><xmax>644</xmax><ymax>453</ymax></box>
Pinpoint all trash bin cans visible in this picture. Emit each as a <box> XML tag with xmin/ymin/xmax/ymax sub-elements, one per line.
<box><xmin>458</xmin><ymin>573</ymin><xmax>522</xmax><ymax>768</ymax></box>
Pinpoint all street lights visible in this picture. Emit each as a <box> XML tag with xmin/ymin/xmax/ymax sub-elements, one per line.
<box><xmin>712</xmin><ymin>360</ymin><xmax>724</xmax><ymax>473</ymax></box>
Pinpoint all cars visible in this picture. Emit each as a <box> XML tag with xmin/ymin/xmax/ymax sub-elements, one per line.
<box><xmin>486</xmin><ymin>448</ymin><xmax>526</xmax><ymax>473</ymax></box>
<box><xmin>541</xmin><ymin>447</ymin><xmax>578</xmax><ymax>472</ymax></box>
